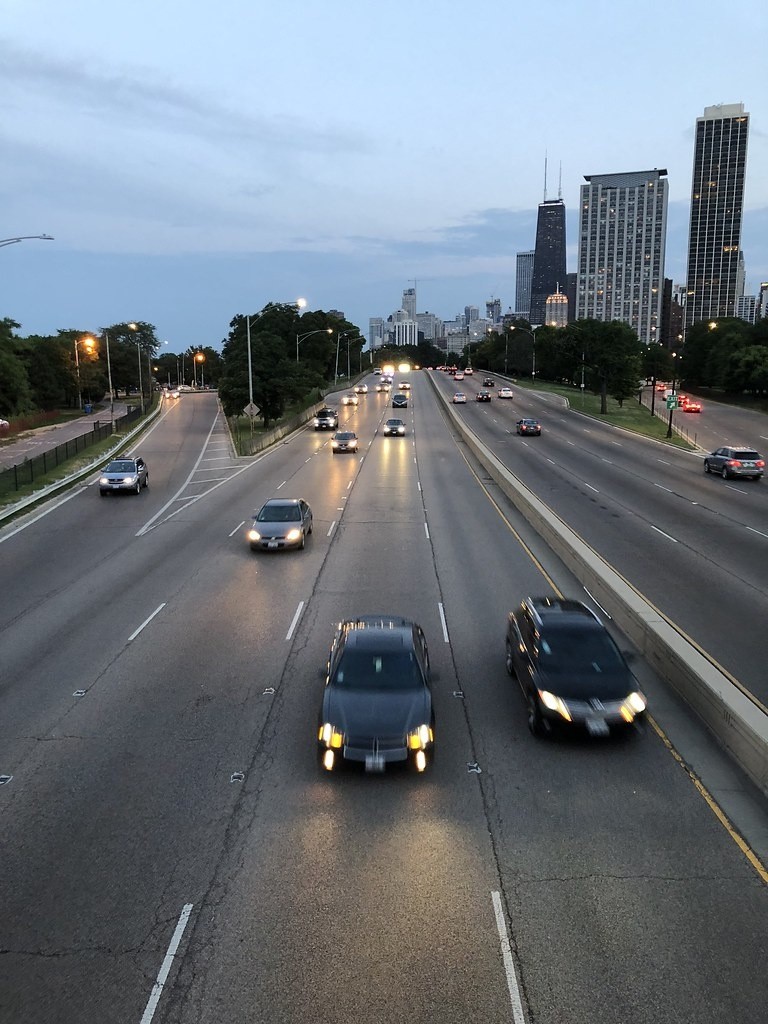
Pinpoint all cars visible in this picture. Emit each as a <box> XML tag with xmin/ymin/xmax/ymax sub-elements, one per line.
<box><xmin>392</xmin><ymin>394</ymin><xmax>409</xmax><ymax>409</ymax></box>
<box><xmin>655</xmin><ymin>384</ymin><xmax>701</xmax><ymax>414</ymax></box>
<box><xmin>342</xmin><ymin>392</ymin><xmax>359</xmax><ymax>405</ymax></box>
<box><xmin>318</xmin><ymin>613</ymin><xmax>441</xmax><ymax>777</ymax></box>
<box><xmin>250</xmin><ymin>497</ymin><xmax>313</xmax><ymax>553</ymax></box>
<box><xmin>331</xmin><ymin>431</ymin><xmax>358</xmax><ymax>453</ymax></box>
<box><xmin>354</xmin><ymin>365</ymin><xmax>513</xmax><ymax>405</ymax></box>
<box><xmin>383</xmin><ymin>418</ymin><xmax>406</xmax><ymax>437</ymax></box>
<box><xmin>155</xmin><ymin>384</ymin><xmax>197</xmax><ymax>399</ymax></box>
<box><xmin>516</xmin><ymin>418</ymin><xmax>542</xmax><ymax>436</ymax></box>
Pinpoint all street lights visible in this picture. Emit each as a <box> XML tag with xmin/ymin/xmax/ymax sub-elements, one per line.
<box><xmin>105</xmin><ymin>323</ymin><xmax>136</xmax><ymax>420</ymax></box>
<box><xmin>551</xmin><ymin>320</ymin><xmax>584</xmax><ymax>408</ymax></box>
<box><xmin>509</xmin><ymin>325</ymin><xmax>535</xmax><ymax>385</ymax></box>
<box><xmin>667</xmin><ymin>319</ymin><xmax>718</xmax><ymax>438</ymax></box>
<box><xmin>488</xmin><ymin>327</ymin><xmax>508</xmax><ymax>378</ymax></box>
<box><xmin>297</xmin><ymin>328</ymin><xmax>333</xmax><ymax>363</ymax></box>
<box><xmin>247</xmin><ymin>298</ymin><xmax>307</xmax><ymax>440</ymax></box>
<box><xmin>74</xmin><ymin>338</ymin><xmax>94</xmax><ymax>410</ymax></box>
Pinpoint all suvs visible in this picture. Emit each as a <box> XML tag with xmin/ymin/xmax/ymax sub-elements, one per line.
<box><xmin>703</xmin><ymin>445</ymin><xmax>765</xmax><ymax>481</ymax></box>
<box><xmin>506</xmin><ymin>595</ymin><xmax>651</xmax><ymax>749</ymax></box>
<box><xmin>313</xmin><ymin>408</ymin><xmax>339</xmax><ymax>431</ymax></box>
<box><xmin>99</xmin><ymin>456</ymin><xmax>148</xmax><ymax>497</ymax></box>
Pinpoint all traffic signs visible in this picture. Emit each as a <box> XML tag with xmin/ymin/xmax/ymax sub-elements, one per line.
<box><xmin>666</xmin><ymin>395</ymin><xmax>678</xmax><ymax>410</ymax></box>
